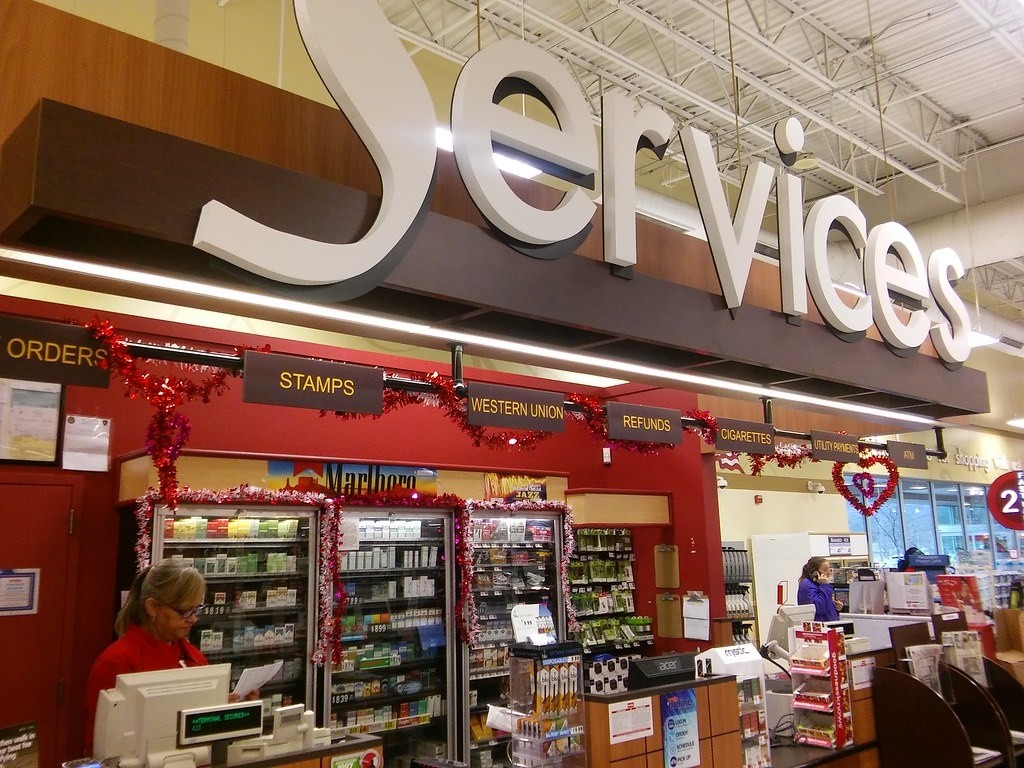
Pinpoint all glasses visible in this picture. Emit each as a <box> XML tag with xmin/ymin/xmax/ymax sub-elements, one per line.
<box><xmin>159</xmin><ymin>600</ymin><xmax>208</xmax><ymax>619</ymax></box>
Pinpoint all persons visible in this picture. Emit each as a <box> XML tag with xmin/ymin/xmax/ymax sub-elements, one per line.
<box><xmin>897</xmin><ymin>548</ymin><xmax>922</xmax><ymax>572</ymax></box>
<box><xmin>85</xmin><ymin>560</ymin><xmax>233</xmax><ymax>754</ymax></box>
<box><xmin>798</xmin><ymin>557</ymin><xmax>841</xmax><ymax>623</ymax></box>
<box><xmin>952</xmin><ymin>578</ymin><xmax>975</xmax><ymax>606</ymax></box>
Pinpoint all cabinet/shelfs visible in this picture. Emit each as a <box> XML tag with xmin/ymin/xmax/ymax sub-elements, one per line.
<box><xmin>788</xmin><ymin>624</ymin><xmax>855</xmax><ymax>750</ymax></box>
<box><xmin>319</xmin><ymin>498</ymin><xmax>466</xmax><ymax>768</ymax></box>
<box><xmin>569</xmin><ymin>531</ymin><xmax>654</xmax><ymax>656</ymax></box>
<box><xmin>937</xmin><ymin>655</ymin><xmax>1024</xmax><ymax>768</ymax></box>
<box><xmin>460</xmin><ymin>510</ymin><xmax>563</xmax><ymax>768</ymax></box>
<box><xmin>510</xmin><ymin>642</ymin><xmax>588</xmax><ymax>768</ymax></box>
<box><xmin>154</xmin><ymin>501</ymin><xmax>317</xmax><ymax>742</ymax></box>
<box><xmin>753</xmin><ymin>532</ymin><xmax>871</xmax><ymax>653</ymax></box>
<box><xmin>872</xmin><ymin>657</ymin><xmax>1015</xmax><ymax>768</ymax></box>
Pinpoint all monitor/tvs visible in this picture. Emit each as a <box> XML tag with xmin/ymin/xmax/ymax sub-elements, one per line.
<box><xmin>95</xmin><ymin>663</ymin><xmax>232</xmax><ymax>751</ymax></box>
<box><xmin>766</xmin><ymin>604</ymin><xmax>817</xmax><ymax>656</ymax></box>
<box><xmin>629</xmin><ymin>651</ymin><xmax>697</xmax><ymax>689</ymax></box>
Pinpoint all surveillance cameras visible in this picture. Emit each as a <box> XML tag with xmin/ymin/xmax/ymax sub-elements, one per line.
<box><xmin>716</xmin><ymin>476</ymin><xmax>728</xmax><ymax>489</ymax></box>
<box><xmin>808</xmin><ymin>480</ymin><xmax>825</xmax><ymax>494</ymax></box>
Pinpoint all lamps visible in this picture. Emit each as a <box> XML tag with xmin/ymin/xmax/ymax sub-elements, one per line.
<box><xmin>962</xmin><ymin>170</ymin><xmax>1001</xmax><ymax>349</ymax></box>
<box><xmin>430</xmin><ymin>2</ymin><xmax>544</xmax><ymax>178</ymax></box>
<box><xmin>830</xmin><ymin>1</ymin><xmax>904</xmax><ymax>297</ymax></box>
<box><xmin>685</xmin><ymin>1</ymin><xmax>742</xmax><ymax>241</ymax></box>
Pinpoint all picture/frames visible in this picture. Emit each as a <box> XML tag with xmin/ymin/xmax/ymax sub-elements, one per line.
<box><xmin>1</xmin><ymin>379</ymin><xmax>67</xmax><ymax>466</ymax></box>
